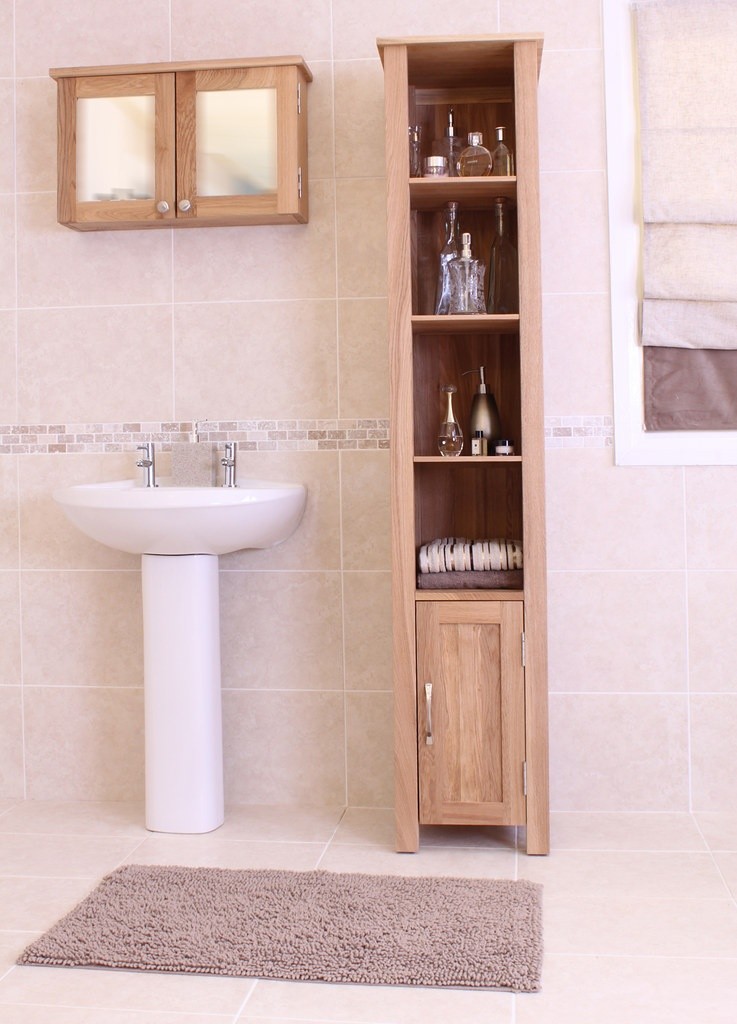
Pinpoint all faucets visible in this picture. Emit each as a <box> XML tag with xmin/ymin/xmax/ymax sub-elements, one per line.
<box><xmin>221</xmin><ymin>441</ymin><xmax>237</xmax><ymax>486</ymax></box>
<box><xmin>136</xmin><ymin>441</ymin><xmax>158</xmax><ymax>489</ymax></box>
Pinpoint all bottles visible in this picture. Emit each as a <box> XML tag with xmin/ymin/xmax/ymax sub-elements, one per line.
<box><xmin>447</xmin><ymin>232</ymin><xmax>487</xmax><ymax>316</ymax></box>
<box><xmin>457</xmin><ymin>132</ymin><xmax>493</xmax><ymax>176</ymax></box>
<box><xmin>409</xmin><ymin>124</ymin><xmax>423</xmax><ymax>178</ymax></box>
<box><xmin>486</xmin><ymin>196</ymin><xmax>516</xmax><ymax>315</ymax></box>
<box><xmin>470</xmin><ymin>430</ymin><xmax>483</xmax><ymax>455</ymax></box>
<box><xmin>493</xmin><ymin>124</ymin><xmax>515</xmax><ymax>176</ymax></box>
<box><xmin>436</xmin><ymin>384</ymin><xmax>465</xmax><ymax>457</ymax></box>
<box><xmin>432</xmin><ymin>201</ymin><xmax>462</xmax><ymax>316</ymax></box>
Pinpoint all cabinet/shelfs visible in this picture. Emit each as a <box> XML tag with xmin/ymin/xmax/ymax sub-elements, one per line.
<box><xmin>376</xmin><ymin>32</ymin><xmax>549</xmax><ymax>856</ymax></box>
<box><xmin>48</xmin><ymin>55</ymin><xmax>312</xmax><ymax>232</ymax></box>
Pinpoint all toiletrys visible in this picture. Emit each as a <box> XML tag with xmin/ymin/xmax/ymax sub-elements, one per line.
<box><xmin>170</xmin><ymin>418</ymin><xmax>218</xmax><ymax>486</ymax></box>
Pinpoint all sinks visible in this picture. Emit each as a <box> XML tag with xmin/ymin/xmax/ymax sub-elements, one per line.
<box><xmin>54</xmin><ymin>480</ymin><xmax>307</xmax><ymax>556</ymax></box>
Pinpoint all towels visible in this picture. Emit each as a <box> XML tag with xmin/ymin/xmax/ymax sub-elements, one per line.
<box><xmin>415</xmin><ymin>571</ymin><xmax>522</xmax><ymax>592</ymax></box>
<box><xmin>633</xmin><ymin>0</ymin><xmax>737</xmax><ymax>435</ymax></box>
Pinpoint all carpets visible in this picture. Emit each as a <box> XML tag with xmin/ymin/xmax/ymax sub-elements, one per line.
<box><xmin>16</xmin><ymin>864</ymin><xmax>543</xmax><ymax>994</ymax></box>
